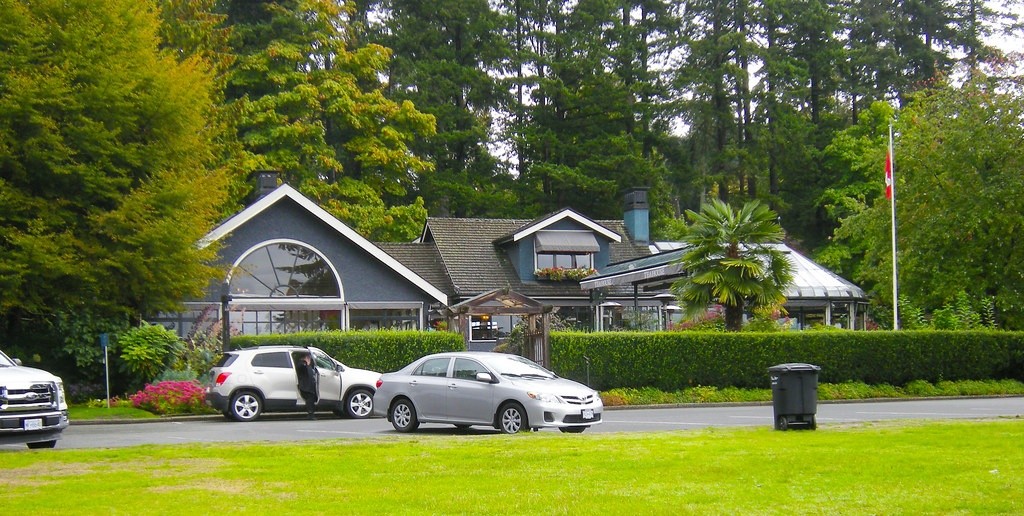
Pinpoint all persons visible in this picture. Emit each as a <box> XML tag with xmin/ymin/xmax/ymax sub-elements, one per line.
<box><xmin>295</xmin><ymin>354</ymin><xmax>318</xmax><ymax>420</ymax></box>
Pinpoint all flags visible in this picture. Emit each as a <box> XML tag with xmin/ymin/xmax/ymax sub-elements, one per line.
<box><xmin>884</xmin><ymin>149</ymin><xmax>891</xmax><ymax>199</ymax></box>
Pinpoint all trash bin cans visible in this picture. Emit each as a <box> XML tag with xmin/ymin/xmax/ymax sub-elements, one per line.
<box><xmin>769</xmin><ymin>362</ymin><xmax>821</xmax><ymax>431</ymax></box>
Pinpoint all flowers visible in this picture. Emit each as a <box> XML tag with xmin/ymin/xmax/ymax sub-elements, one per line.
<box><xmin>533</xmin><ymin>265</ymin><xmax>597</xmax><ymax>282</ymax></box>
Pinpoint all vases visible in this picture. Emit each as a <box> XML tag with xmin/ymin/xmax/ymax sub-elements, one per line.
<box><xmin>535</xmin><ymin>273</ymin><xmax>568</xmax><ymax>280</ymax></box>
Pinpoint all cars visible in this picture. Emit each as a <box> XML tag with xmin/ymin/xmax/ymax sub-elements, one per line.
<box><xmin>373</xmin><ymin>351</ymin><xmax>604</xmax><ymax>434</ymax></box>
<box><xmin>0</xmin><ymin>350</ymin><xmax>69</xmax><ymax>449</ymax></box>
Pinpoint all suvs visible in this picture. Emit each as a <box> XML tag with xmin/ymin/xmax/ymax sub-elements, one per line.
<box><xmin>206</xmin><ymin>345</ymin><xmax>382</xmax><ymax>422</ymax></box>
